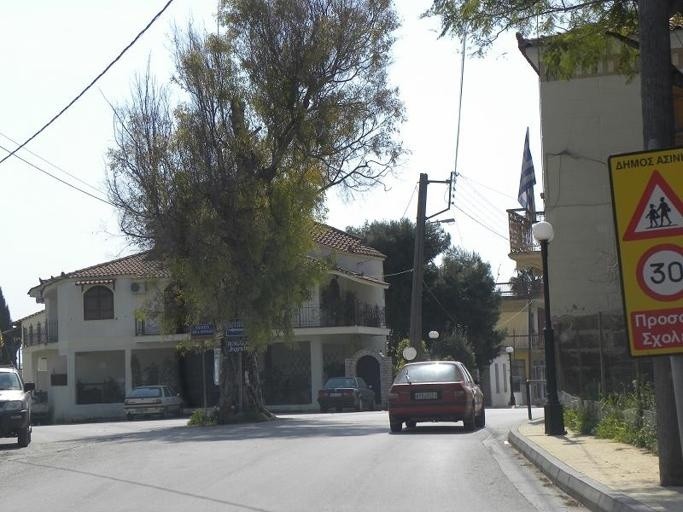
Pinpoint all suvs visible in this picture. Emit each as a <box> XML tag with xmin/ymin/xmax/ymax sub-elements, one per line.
<box><xmin>0</xmin><ymin>366</ymin><xmax>38</xmax><ymax>450</ymax></box>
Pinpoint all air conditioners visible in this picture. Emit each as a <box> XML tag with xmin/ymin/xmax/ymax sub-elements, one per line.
<box><xmin>129</xmin><ymin>281</ymin><xmax>146</xmax><ymax>294</ymax></box>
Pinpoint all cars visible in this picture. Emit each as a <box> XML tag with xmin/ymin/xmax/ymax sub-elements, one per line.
<box><xmin>386</xmin><ymin>359</ymin><xmax>486</xmax><ymax>437</ymax></box>
<box><xmin>315</xmin><ymin>374</ymin><xmax>379</xmax><ymax>415</ymax></box>
<box><xmin>29</xmin><ymin>395</ymin><xmax>51</xmax><ymax>428</ymax></box>
<box><xmin>119</xmin><ymin>383</ymin><xmax>185</xmax><ymax>419</ymax></box>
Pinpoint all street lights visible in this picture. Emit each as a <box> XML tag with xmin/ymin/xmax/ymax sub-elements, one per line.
<box><xmin>405</xmin><ymin>217</ymin><xmax>455</xmax><ymax>361</ymax></box>
<box><xmin>427</xmin><ymin>330</ymin><xmax>439</xmax><ymax>361</ymax></box>
<box><xmin>530</xmin><ymin>218</ymin><xmax>569</xmax><ymax>438</ymax></box>
<box><xmin>504</xmin><ymin>345</ymin><xmax>517</xmax><ymax>406</ymax></box>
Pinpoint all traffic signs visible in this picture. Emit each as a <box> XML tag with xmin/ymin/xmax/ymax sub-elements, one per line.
<box><xmin>633</xmin><ymin>241</ymin><xmax>682</xmax><ymax>303</ymax></box>
<box><xmin>227</xmin><ymin>339</ymin><xmax>250</xmax><ymax>353</ymax></box>
<box><xmin>190</xmin><ymin>322</ymin><xmax>217</xmax><ymax>339</ymax></box>
<box><xmin>223</xmin><ymin>320</ymin><xmax>250</xmax><ymax>337</ymax></box>
